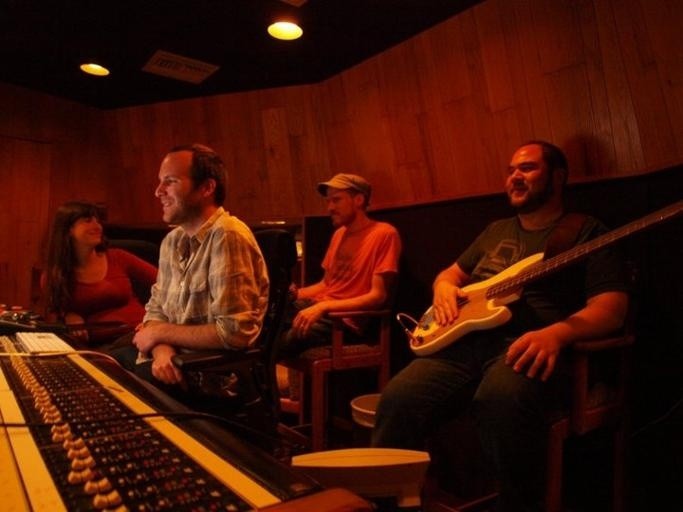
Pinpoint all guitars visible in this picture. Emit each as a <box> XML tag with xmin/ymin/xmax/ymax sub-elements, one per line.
<box><xmin>408</xmin><ymin>202</ymin><xmax>683</xmax><ymax>356</ymax></box>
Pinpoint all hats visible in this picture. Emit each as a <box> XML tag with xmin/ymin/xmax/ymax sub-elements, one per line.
<box><xmin>315</xmin><ymin>173</ymin><xmax>371</xmax><ymax>199</ymax></box>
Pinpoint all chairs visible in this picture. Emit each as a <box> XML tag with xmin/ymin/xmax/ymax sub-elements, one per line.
<box><xmin>270</xmin><ymin>305</ymin><xmax>399</xmax><ymax>452</ymax></box>
<box><xmin>169</xmin><ymin>227</ymin><xmax>294</xmax><ymax>478</ymax></box>
<box><xmin>424</xmin><ymin>259</ymin><xmax>636</xmax><ymax>511</ymax></box>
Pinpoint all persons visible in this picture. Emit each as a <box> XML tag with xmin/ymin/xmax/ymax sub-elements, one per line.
<box><xmin>370</xmin><ymin>138</ymin><xmax>642</xmax><ymax>510</ymax></box>
<box><xmin>133</xmin><ymin>142</ymin><xmax>278</xmax><ymax>401</ymax></box>
<box><xmin>28</xmin><ymin>202</ymin><xmax>160</xmax><ymax>350</ymax></box>
<box><xmin>279</xmin><ymin>167</ymin><xmax>405</xmax><ymax>396</ymax></box>
